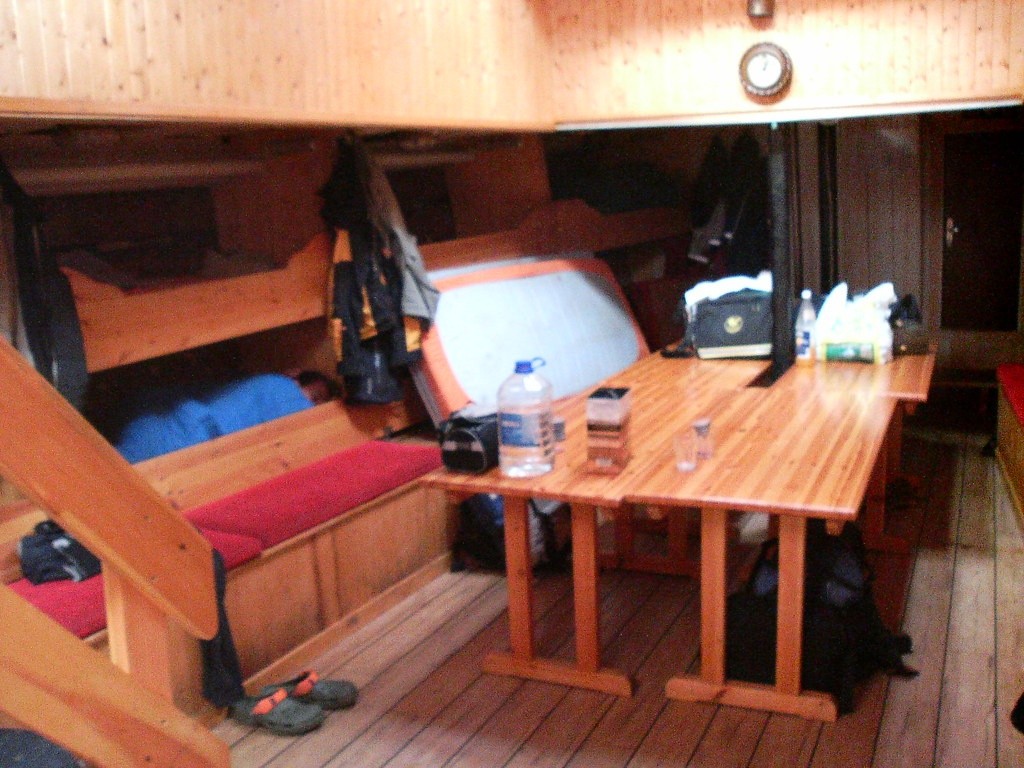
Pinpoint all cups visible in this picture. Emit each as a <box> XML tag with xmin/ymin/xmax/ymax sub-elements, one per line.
<box><xmin>673</xmin><ymin>435</ymin><xmax>698</xmax><ymax>471</ymax></box>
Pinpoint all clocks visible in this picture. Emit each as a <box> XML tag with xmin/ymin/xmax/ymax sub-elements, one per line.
<box><xmin>740</xmin><ymin>41</ymin><xmax>794</xmax><ymax>99</ymax></box>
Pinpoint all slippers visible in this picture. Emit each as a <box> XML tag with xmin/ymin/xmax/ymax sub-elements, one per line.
<box><xmin>261</xmin><ymin>670</ymin><xmax>356</xmax><ymax>710</ymax></box>
<box><xmin>233</xmin><ymin>687</ymin><xmax>322</xmax><ymax>737</ymax></box>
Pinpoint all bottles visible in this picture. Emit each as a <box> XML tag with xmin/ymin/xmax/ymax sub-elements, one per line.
<box><xmin>795</xmin><ymin>288</ymin><xmax>816</xmax><ymax>362</ymax></box>
<box><xmin>496</xmin><ymin>356</ymin><xmax>559</xmax><ymax>478</ymax></box>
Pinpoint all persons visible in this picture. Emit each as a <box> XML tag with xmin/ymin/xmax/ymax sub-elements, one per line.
<box><xmin>116</xmin><ymin>370</ymin><xmax>331</xmax><ymax>468</ymax></box>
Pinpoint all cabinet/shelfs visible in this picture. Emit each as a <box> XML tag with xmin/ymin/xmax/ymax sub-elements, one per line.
<box><xmin>3</xmin><ymin>118</ymin><xmax>687</xmax><ymax>373</ymax></box>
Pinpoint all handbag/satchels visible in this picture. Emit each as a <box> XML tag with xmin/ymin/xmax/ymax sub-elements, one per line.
<box><xmin>724</xmin><ymin>520</ymin><xmax>913</xmax><ymax>713</ymax></box>
<box><xmin>810</xmin><ymin>278</ymin><xmax>897</xmax><ymax>364</ymax></box>
<box><xmin>453</xmin><ymin>494</ymin><xmax>551</xmax><ymax>574</ymax></box>
<box><xmin>437</xmin><ymin>409</ymin><xmax>500</xmax><ymax>475</ymax></box>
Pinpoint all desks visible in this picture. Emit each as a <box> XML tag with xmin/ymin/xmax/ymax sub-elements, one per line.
<box><xmin>425</xmin><ymin>337</ymin><xmax>939</xmax><ymax>724</ymax></box>
<box><xmin>8</xmin><ymin>419</ymin><xmax>451</xmax><ymax>713</ymax></box>
